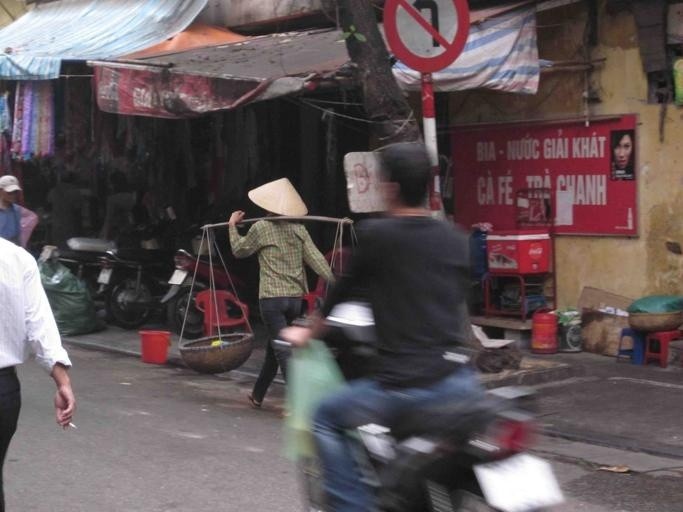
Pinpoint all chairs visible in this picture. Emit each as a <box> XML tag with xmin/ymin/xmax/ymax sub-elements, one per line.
<box><xmin>195</xmin><ymin>290</ymin><xmax>251</xmax><ymax>341</ymax></box>
<box><xmin>300</xmin><ymin>246</ymin><xmax>352</xmax><ymax>318</ymax></box>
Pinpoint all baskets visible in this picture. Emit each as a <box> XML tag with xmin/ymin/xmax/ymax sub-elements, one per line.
<box><xmin>179</xmin><ymin>332</ymin><xmax>255</xmax><ymax>375</ymax></box>
<box><xmin>628</xmin><ymin>309</ymin><xmax>682</xmax><ymax>333</ymax></box>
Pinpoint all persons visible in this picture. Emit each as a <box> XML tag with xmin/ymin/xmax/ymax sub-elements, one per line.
<box><xmin>0</xmin><ymin>237</ymin><xmax>76</xmax><ymax>512</ymax></box>
<box><xmin>0</xmin><ymin>175</ymin><xmax>22</xmax><ymax>245</ymax></box>
<box><xmin>611</xmin><ymin>131</ymin><xmax>633</xmax><ymax>175</ymax></box>
<box><xmin>229</xmin><ymin>176</ymin><xmax>336</xmax><ymax>410</ymax></box>
<box><xmin>279</xmin><ymin>141</ymin><xmax>481</xmax><ymax>512</ymax></box>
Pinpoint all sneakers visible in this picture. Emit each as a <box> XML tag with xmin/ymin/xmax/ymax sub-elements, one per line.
<box><xmin>246</xmin><ymin>390</ymin><xmax>263</xmax><ymax>410</ymax></box>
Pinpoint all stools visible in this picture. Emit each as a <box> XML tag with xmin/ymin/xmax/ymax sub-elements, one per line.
<box><xmin>616</xmin><ymin>327</ymin><xmax>683</xmax><ymax>368</ymax></box>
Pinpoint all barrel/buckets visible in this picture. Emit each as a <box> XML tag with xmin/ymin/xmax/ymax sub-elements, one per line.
<box><xmin>531</xmin><ymin>306</ymin><xmax>558</xmax><ymax>354</ymax></box>
<box><xmin>559</xmin><ymin>318</ymin><xmax>583</xmax><ymax>354</ymax></box>
<box><xmin>138</xmin><ymin>330</ymin><xmax>172</xmax><ymax>364</ymax></box>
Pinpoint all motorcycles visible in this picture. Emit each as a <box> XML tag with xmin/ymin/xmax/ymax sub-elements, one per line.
<box><xmin>160</xmin><ymin>243</ymin><xmax>269</xmax><ymax>340</ymax></box>
<box><xmin>270</xmin><ymin>281</ymin><xmax>566</xmax><ymax>512</ymax></box>
<box><xmin>93</xmin><ymin>212</ymin><xmax>249</xmax><ymax>330</ymax></box>
<box><xmin>43</xmin><ymin>206</ymin><xmax>179</xmax><ymax>327</ymax></box>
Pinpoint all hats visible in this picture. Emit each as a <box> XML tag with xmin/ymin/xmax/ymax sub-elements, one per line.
<box><xmin>0</xmin><ymin>175</ymin><xmax>23</xmax><ymax>193</ymax></box>
<box><xmin>247</xmin><ymin>177</ymin><xmax>309</xmax><ymax>217</ymax></box>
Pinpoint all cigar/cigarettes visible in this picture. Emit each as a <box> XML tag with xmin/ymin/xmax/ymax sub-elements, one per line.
<box><xmin>68</xmin><ymin>422</ymin><xmax>77</xmax><ymax>430</ymax></box>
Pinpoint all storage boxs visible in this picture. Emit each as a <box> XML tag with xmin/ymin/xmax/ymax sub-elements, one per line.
<box><xmin>486</xmin><ymin>227</ymin><xmax>551</xmax><ymax>275</ymax></box>
